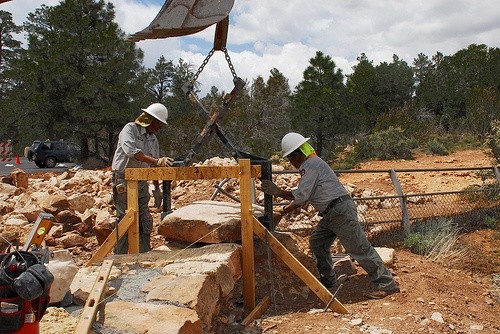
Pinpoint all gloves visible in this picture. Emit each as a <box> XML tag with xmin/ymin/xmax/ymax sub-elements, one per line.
<box><xmin>274</xmin><ymin>205</ymin><xmax>287</xmax><ymax>216</ymax></box>
<box><xmin>154</xmin><ymin>156</ymin><xmax>174</xmax><ymax>167</ymax></box>
<box><xmin>260</xmin><ymin>179</ymin><xmax>281</xmax><ymax>198</ymax></box>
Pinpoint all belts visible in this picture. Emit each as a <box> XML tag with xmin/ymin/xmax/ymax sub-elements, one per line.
<box><xmin>321</xmin><ymin>195</ymin><xmax>351</xmax><ymax>215</ymax></box>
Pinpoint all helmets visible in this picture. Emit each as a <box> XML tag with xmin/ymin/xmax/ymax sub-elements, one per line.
<box><xmin>282</xmin><ymin>132</ymin><xmax>310</xmax><ymax>158</ymax></box>
<box><xmin>141</xmin><ymin>103</ymin><xmax>168</xmax><ymax>125</ymax></box>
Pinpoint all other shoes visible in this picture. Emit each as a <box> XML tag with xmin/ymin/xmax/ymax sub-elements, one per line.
<box><xmin>366</xmin><ymin>287</ymin><xmax>393</xmax><ymax>299</ymax></box>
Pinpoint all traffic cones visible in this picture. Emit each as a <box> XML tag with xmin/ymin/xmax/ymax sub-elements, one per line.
<box><xmin>16</xmin><ymin>155</ymin><xmax>20</xmax><ymax>164</ymax></box>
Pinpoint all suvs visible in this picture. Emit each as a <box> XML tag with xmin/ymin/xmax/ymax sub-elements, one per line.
<box><xmin>24</xmin><ymin>140</ymin><xmax>94</xmax><ymax>169</ymax></box>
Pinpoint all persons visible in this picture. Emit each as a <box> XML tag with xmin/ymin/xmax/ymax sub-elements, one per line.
<box><xmin>106</xmin><ymin>103</ymin><xmax>174</xmax><ymax>252</ymax></box>
<box><xmin>260</xmin><ymin>132</ymin><xmax>400</xmax><ymax>299</ymax></box>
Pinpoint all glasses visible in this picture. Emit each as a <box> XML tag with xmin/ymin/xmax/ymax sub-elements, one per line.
<box><xmin>287</xmin><ymin>149</ymin><xmax>301</xmax><ymax>161</ymax></box>
<box><xmin>154</xmin><ymin>118</ymin><xmax>165</xmax><ymax>127</ymax></box>
